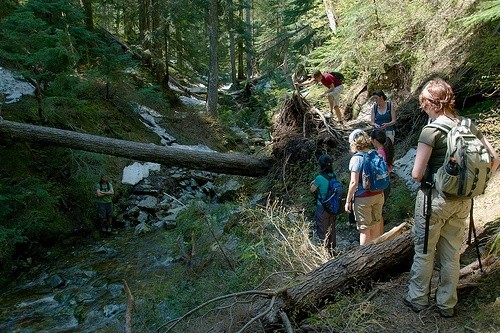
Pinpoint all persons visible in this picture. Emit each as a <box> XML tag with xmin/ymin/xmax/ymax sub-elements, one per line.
<box><xmin>344</xmin><ymin>89</ymin><xmax>397</xmax><ymax>245</ymax></box>
<box><xmin>310</xmin><ymin>154</ymin><xmax>338</xmax><ymax>256</ymax></box>
<box><xmin>401</xmin><ymin>78</ymin><xmax>499</xmax><ymax>316</ymax></box>
<box><xmin>96</xmin><ymin>174</ymin><xmax>115</xmax><ymax>232</ymax></box>
<box><xmin>293</xmin><ymin>70</ymin><xmax>345</xmax><ymax>126</ymax></box>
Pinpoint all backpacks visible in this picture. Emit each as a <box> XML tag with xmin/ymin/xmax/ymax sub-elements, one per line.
<box><xmin>354</xmin><ymin>149</ymin><xmax>390</xmax><ymax>192</ymax></box>
<box><xmin>313</xmin><ymin>172</ymin><xmax>348</xmax><ymax>216</ymax></box>
<box><xmin>324</xmin><ymin>72</ymin><xmax>344</xmax><ymax>82</ymax></box>
<box><xmin>422</xmin><ymin>115</ymin><xmax>492</xmax><ymax>199</ymax></box>
<box><xmin>383</xmin><ymin>136</ymin><xmax>394</xmax><ymax>165</ymax></box>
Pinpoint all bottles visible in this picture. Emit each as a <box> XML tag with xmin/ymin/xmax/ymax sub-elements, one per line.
<box><xmin>444</xmin><ymin>158</ymin><xmax>459</xmax><ymax>176</ymax></box>
<box><xmin>364</xmin><ymin>170</ymin><xmax>369</xmax><ymax>186</ymax></box>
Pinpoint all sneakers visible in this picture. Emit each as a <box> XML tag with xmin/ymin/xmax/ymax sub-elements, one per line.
<box><xmin>325</xmin><ymin>112</ymin><xmax>334</xmax><ymax>117</ymax></box>
<box><xmin>402</xmin><ymin>298</ymin><xmax>427</xmax><ymax>315</ymax></box>
<box><xmin>434</xmin><ymin>305</ymin><xmax>454</xmax><ymax>318</ymax></box>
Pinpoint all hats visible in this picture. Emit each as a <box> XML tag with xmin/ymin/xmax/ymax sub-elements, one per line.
<box><xmin>369</xmin><ymin>129</ymin><xmax>386</xmax><ymax>143</ymax></box>
<box><xmin>314</xmin><ymin>154</ymin><xmax>332</xmax><ymax>165</ymax></box>
<box><xmin>349</xmin><ymin>129</ymin><xmax>364</xmax><ymax>143</ymax></box>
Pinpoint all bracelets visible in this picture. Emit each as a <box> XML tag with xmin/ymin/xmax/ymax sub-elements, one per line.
<box><xmin>346</xmin><ymin>201</ymin><xmax>351</xmax><ymax>203</ymax></box>
